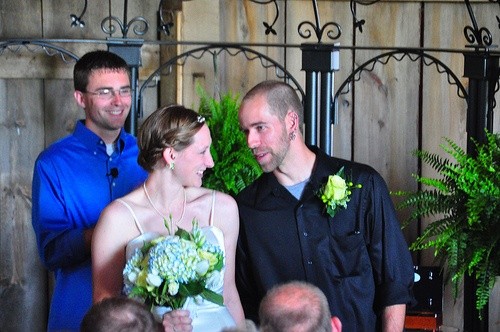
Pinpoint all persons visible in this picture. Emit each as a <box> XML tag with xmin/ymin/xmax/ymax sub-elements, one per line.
<box><xmin>234</xmin><ymin>79</ymin><xmax>415</xmax><ymax>332</ymax></box>
<box><xmin>80</xmin><ymin>281</ymin><xmax>342</xmax><ymax>332</ymax></box>
<box><xmin>31</xmin><ymin>48</ymin><xmax>150</xmax><ymax>332</ymax></box>
<box><xmin>90</xmin><ymin>105</ymin><xmax>247</xmax><ymax>332</ymax></box>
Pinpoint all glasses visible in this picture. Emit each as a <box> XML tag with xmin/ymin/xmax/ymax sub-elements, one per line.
<box><xmin>80</xmin><ymin>85</ymin><xmax>132</xmax><ymax>97</ymax></box>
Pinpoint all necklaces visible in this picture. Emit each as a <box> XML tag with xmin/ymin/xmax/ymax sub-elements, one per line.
<box><xmin>143</xmin><ymin>179</ymin><xmax>186</xmax><ymax>224</ymax></box>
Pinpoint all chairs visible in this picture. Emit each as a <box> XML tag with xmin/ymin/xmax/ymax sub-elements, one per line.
<box><xmin>403</xmin><ymin>266</ymin><xmax>443</xmax><ymax>332</ymax></box>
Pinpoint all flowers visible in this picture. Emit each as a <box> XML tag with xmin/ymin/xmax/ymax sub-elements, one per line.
<box><xmin>122</xmin><ymin>209</ymin><xmax>225</xmax><ymax>310</ymax></box>
<box><xmin>312</xmin><ymin>166</ymin><xmax>362</xmax><ymax>218</ymax></box>
<box><xmin>390</xmin><ymin>127</ymin><xmax>500</xmax><ymax>321</ymax></box>
